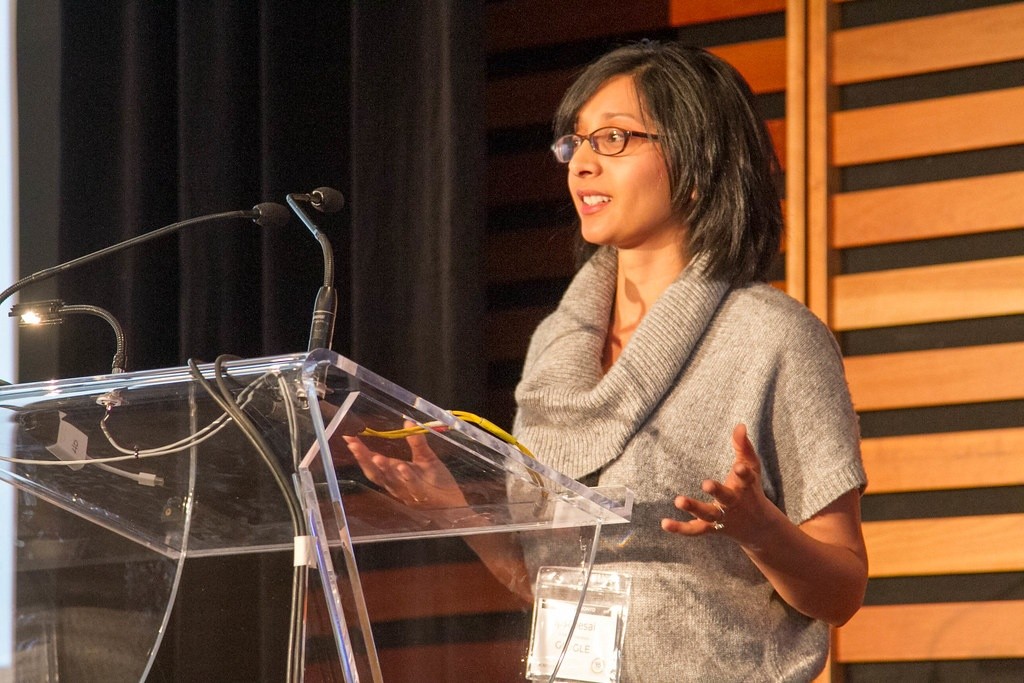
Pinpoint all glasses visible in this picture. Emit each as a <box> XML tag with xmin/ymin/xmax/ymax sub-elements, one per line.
<box><xmin>550</xmin><ymin>126</ymin><xmax>668</xmax><ymax>163</ymax></box>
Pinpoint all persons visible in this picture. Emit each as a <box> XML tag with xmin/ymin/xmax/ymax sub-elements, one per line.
<box><xmin>344</xmin><ymin>39</ymin><xmax>868</xmax><ymax>683</ymax></box>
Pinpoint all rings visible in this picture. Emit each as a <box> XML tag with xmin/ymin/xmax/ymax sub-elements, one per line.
<box><xmin>712</xmin><ymin>503</ymin><xmax>725</xmax><ymax>530</ymax></box>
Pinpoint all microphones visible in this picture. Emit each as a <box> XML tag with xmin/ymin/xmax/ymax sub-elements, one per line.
<box><xmin>0</xmin><ymin>202</ymin><xmax>290</xmax><ymax>305</ymax></box>
<box><xmin>284</xmin><ymin>188</ymin><xmax>344</xmax><ymax>398</ymax></box>
